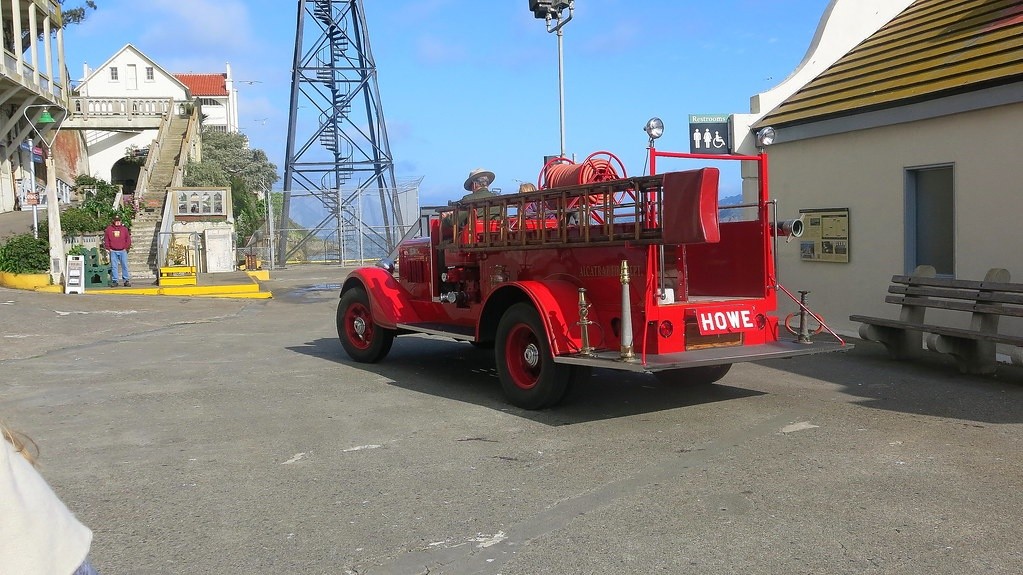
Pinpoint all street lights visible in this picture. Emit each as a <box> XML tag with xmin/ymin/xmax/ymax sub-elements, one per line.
<box><xmin>528</xmin><ymin>0</ymin><xmax>574</xmax><ymax>164</ymax></box>
<box><xmin>24</xmin><ymin>103</ymin><xmax>68</xmax><ymax>282</ymax></box>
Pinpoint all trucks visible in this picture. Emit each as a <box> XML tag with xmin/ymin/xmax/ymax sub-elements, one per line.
<box><xmin>334</xmin><ymin>118</ymin><xmax>859</xmax><ymax>408</ymax></box>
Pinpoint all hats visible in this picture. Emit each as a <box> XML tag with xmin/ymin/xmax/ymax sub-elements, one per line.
<box><xmin>113</xmin><ymin>216</ymin><xmax>120</xmax><ymax>221</ymax></box>
<box><xmin>464</xmin><ymin>169</ymin><xmax>496</xmax><ymax>191</ymax></box>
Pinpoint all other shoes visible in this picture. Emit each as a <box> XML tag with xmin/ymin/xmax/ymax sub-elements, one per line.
<box><xmin>124</xmin><ymin>282</ymin><xmax>131</xmax><ymax>287</ymax></box>
<box><xmin>111</xmin><ymin>281</ymin><xmax>118</xmax><ymax>287</ymax></box>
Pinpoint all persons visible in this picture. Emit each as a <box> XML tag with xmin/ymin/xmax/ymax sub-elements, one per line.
<box><xmin>438</xmin><ymin>168</ymin><xmax>559</xmax><ymax>295</ymax></box>
<box><xmin>105</xmin><ymin>215</ymin><xmax>133</xmax><ymax>287</ymax></box>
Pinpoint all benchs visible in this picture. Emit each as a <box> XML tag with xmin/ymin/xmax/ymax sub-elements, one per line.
<box><xmin>850</xmin><ymin>264</ymin><xmax>1023</xmax><ymax>378</ymax></box>
<box><xmin>444</xmin><ymin>208</ymin><xmax>566</xmax><ymax>267</ymax></box>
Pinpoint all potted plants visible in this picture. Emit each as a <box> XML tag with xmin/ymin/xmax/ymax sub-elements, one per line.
<box><xmin>167</xmin><ymin>236</ymin><xmax>190</xmax><ymax>276</ymax></box>
